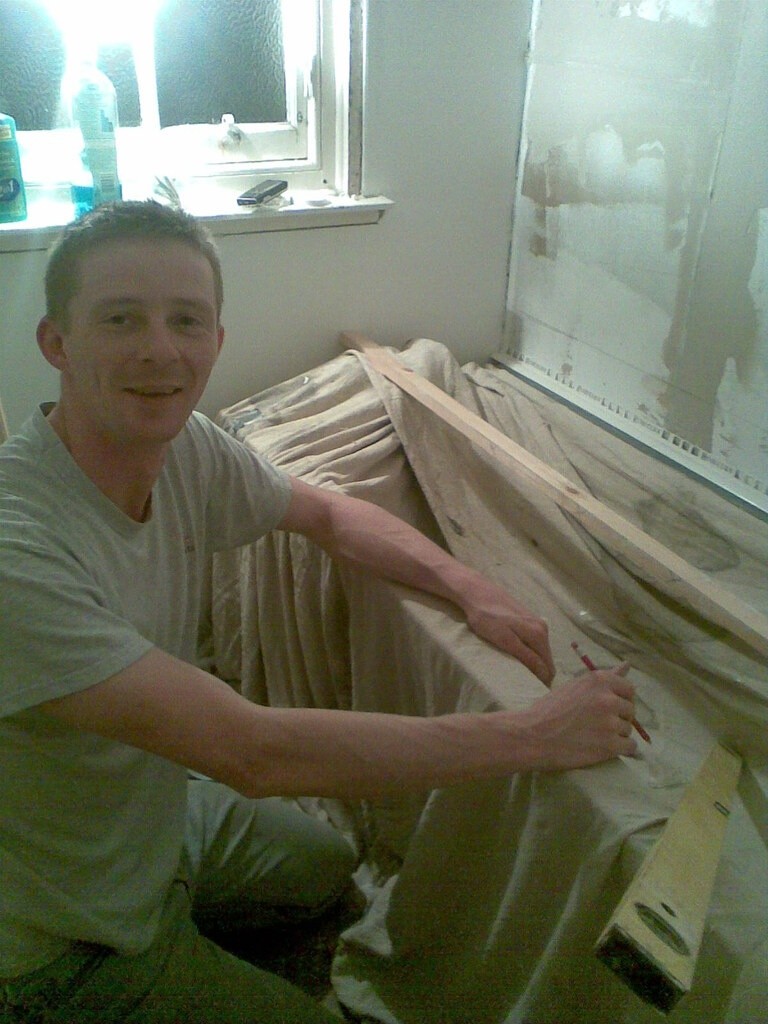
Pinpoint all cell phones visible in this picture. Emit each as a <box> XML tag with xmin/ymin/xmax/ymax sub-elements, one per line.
<box><xmin>237</xmin><ymin>179</ymin><xmax>287</xmax><ymax>206</ymax></box>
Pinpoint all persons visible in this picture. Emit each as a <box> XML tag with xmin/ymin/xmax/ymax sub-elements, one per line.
<box><xmin>0</xmin><ymin>201</ymin><xmax>636</xmax><ymax>1024</ymax></box>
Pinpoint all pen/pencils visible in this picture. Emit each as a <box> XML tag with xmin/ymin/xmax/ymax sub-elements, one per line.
<box><xmin>570</xmin><ymin>640</ymin><xmax>653</xmax><ymax>745</ymax></box>
